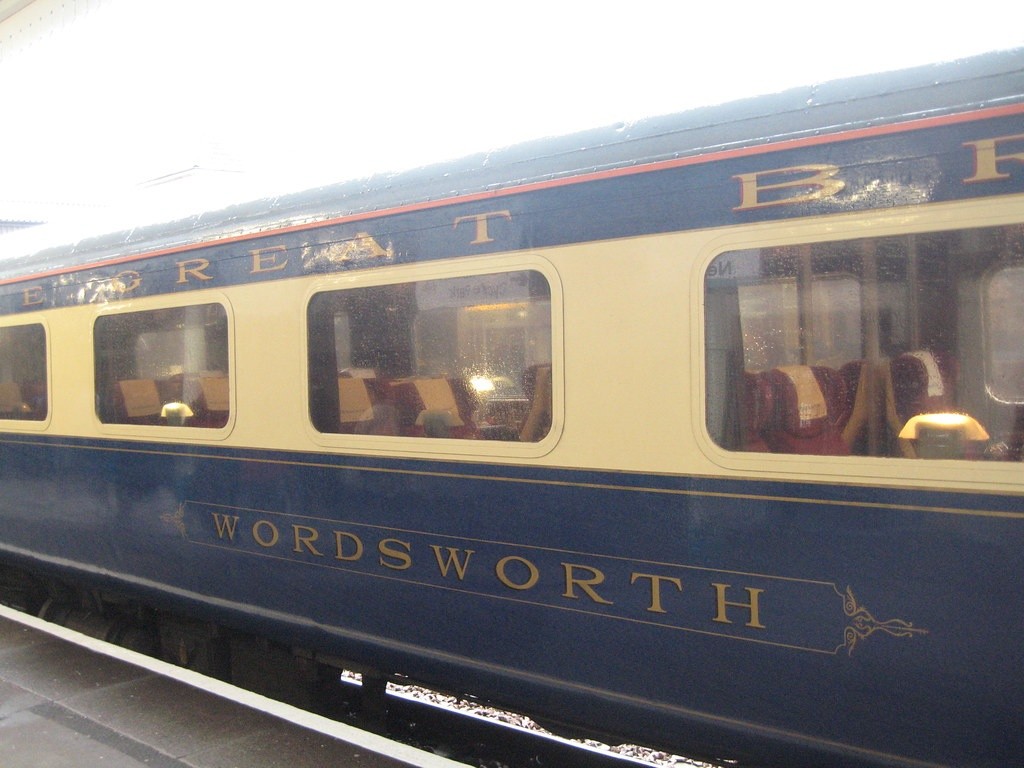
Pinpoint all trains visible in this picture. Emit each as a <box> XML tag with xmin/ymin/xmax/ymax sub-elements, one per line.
<box><xmin>1</xmin><ymin>48</ymin><xmax>1024</xmax><ymax>768</ymax></box>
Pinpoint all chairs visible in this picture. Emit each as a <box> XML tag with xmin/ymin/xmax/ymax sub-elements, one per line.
<box><xmin>1</xmin><ymin>378</ymin><xmax>47</xmax><ymax>421</ymax></box>
<box><xmin>111</xmin><ymin>369</ymin><xmax>230</xmax><ymax>427</ymax></box>
<box><xmin>338</xmin><ymin>360</ymin><xmax>552</xmax><ymax>442</ymax></box>
<box><xmin>734</xmin><ymin>350</ymin><xmax>1023</xmax><ymax>462</ymax></box>
